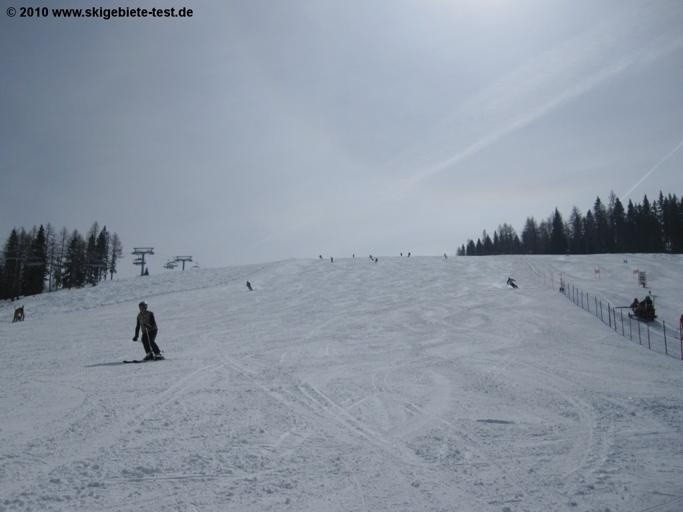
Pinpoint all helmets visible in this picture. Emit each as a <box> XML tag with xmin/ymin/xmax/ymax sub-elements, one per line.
<box><xmin>139</xmin><ymin>302</ymin><xmax>148</xmax><ymax>310</ymax></box>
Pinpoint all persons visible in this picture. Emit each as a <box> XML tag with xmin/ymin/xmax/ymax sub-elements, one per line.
<box><xmin>246</xmin><ymin>280</ymin><xmax>253</xmax><ymax>291</ymax></box>
<box><xmin>13</xmin><ymin>304</ymin><xmax>25</xmax><ymax>321</ymax></box>
<box><xmin>507</xmin><ymin>276</ymin><xmax>518</xmax><ymax>288</ymax></box>
<box><xmin>630</xmin><ymin>295</ymin><xmax>656</xmax><ymax>322</ymax></box>
<box><xmin>133</xmin><ymin>300</ymin><xmax>164</xmax><ymax>361</ymax></box>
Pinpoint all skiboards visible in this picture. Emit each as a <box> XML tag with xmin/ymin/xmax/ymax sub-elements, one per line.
<box><xmin>124</xmin><ymin>356</ymin><xmax>167</xmax><ymax>362</ymax></box>
